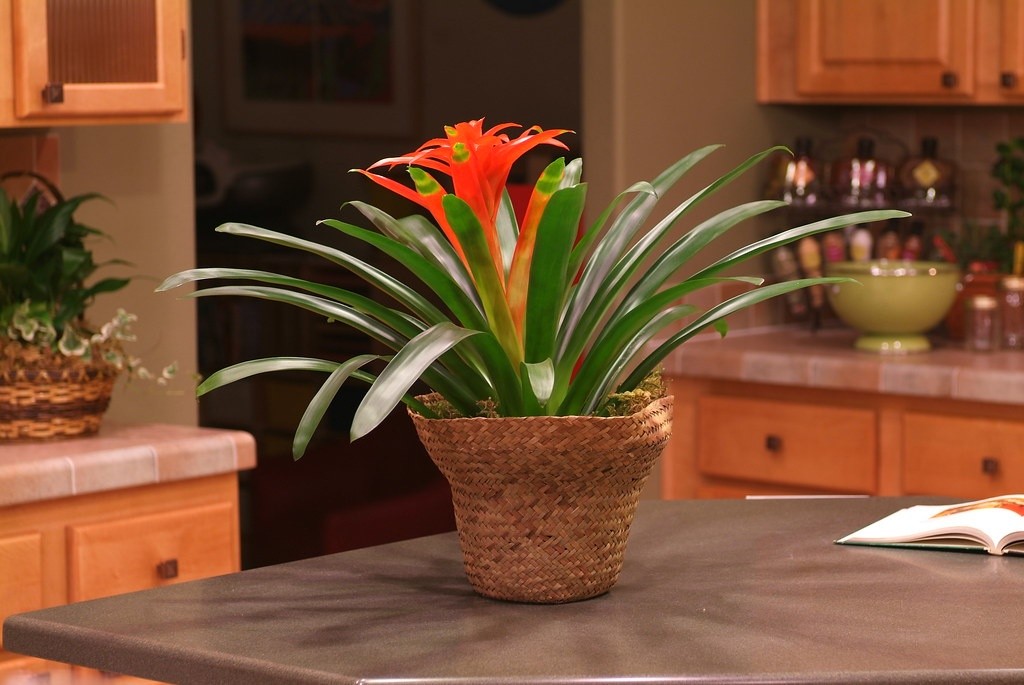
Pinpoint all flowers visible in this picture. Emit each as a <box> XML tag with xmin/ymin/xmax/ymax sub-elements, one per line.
<box><xmin>154</xmin><ymin>122</ymin><xmax>921</xmax><ymax>447</ymax></box>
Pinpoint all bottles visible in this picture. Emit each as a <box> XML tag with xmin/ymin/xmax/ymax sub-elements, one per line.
<box><xmin>947</xmin><ymin>263</ymin><xmax>1024</xmax><ymax>356</ymax></box>
<box><xmin>772</xmin><ymin>128</ymin><xmax>952</xmax><ymax>328</ymax></box>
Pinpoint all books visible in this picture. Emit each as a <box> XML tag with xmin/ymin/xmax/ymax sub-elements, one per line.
<box><xmin>832</xmin><ymin>493</ymin><xmax>1024</xmax><ymax>556</ymax></box>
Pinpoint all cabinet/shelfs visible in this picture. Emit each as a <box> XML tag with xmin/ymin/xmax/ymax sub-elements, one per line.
<box><xmin>2</xmin><ymin>421</ymin><xmax>258</xmax><ymax>685</ymax></box>
<box><xmin>1</xmin><ymin>0</ymin><xmax>196</xmax><ymax>133</ymax></box>
<box><xmin>754</xmin><ymin>3</ymin><xmax>1024</xmax><ymax>109</ymax></box>
<box><xmin>652</xmin><ymin>314</ymin><xmax>1024</xmax><ymax>508</ymax></box>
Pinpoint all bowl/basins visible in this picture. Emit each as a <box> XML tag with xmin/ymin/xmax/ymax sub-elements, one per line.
<box><xmin>824</xmin><ymin>256</ymin><xmax>959</xmax><ymax>353</ymax></box>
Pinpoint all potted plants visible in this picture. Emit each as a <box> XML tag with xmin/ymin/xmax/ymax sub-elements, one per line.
<box><xmin>0</xmin><ymin>177</ymin><xmax>143</xmax><ymax>446</ymax></box>
<box><xmin>400</xmin><ymin>379</ymin><xmax>681</xmax><ymax>602</ymax></box>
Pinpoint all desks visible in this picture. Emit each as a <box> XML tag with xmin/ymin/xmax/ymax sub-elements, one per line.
<box><xmin>6</xmin><ymin>478</ymin><xmax>1024</xmax><ymax>685</ymax></box>
